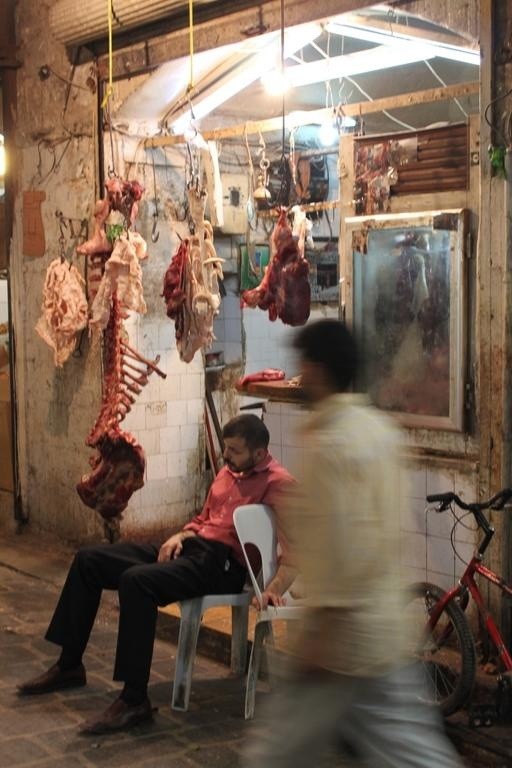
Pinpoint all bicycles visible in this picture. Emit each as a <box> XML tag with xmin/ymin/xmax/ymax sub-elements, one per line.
<box><xmin>400</xmin><ymin>488</ymin><xmax>512</xmax><ymax>717</ymax></box>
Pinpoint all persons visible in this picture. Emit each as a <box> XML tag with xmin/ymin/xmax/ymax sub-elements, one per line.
<box><xmin>15</xmin><ymin>414</ymin><xmax>304</xmax><ymax>734</ymax></box>
<box><xmin>238</xmin><ymin>318</ymin><xmax>472</xmax><ymax>768</ymax></box>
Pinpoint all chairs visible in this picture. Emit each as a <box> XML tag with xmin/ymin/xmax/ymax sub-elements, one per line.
<box><xmin>171</xmin><ymin>502</ymin><xmax>307</xmax><ymax>722</ymax></box>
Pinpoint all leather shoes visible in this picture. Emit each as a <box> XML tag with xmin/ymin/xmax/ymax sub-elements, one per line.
<box><xmin>78</xmin><ymin>698</ymin><xmax>154</xmax><ymax>735</ymax></box>
<box><xmin>17</xmin><ymin>664</ymin><xmax>86</xmax><ymax>695</ymax></box>
